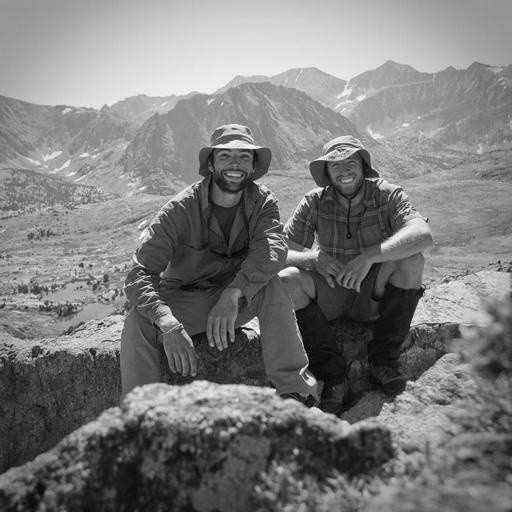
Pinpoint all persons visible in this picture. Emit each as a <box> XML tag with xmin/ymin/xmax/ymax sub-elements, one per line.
<box><xmin>118</xmin><ymin>123</ymin><xmax>320</xmax><ymax>408</ymax></box>
<box><xmin>279</xmin><ymin>134</ymin><xmax>434</xmax><ymax>414</ymax></box>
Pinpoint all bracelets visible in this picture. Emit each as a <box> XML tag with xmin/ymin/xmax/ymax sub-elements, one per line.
<box><xmin>161</xmin><ymin>322</ymin><xmax>185</xmax><ymax>337</ymax></box>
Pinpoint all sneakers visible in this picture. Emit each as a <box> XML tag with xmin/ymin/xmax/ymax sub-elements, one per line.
<box><xmin>367</xmin><ymin>361</ymin><xmax>409</xmax><ymax>401</ymax></box>
<box><xmin>269</xmin><ymin>372</ymin><xmax>348</xmax><ymax>416</ymax></box>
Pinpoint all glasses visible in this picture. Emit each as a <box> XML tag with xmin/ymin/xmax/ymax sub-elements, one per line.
<box><xmin>207</xmin><ymin>217</ymin><xmax>248</xmax><ymax>258</ymax></box>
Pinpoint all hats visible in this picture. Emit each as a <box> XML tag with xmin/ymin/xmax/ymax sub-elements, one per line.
<box><xmin>199</xmin><ymin>123</ymin><xmax>272</xmax><ymax>185</ymax></box>
<box><xmin>309</xmin><ymin>135</ymin><xmax>380</xmax><ymax>187</ymax></box>
